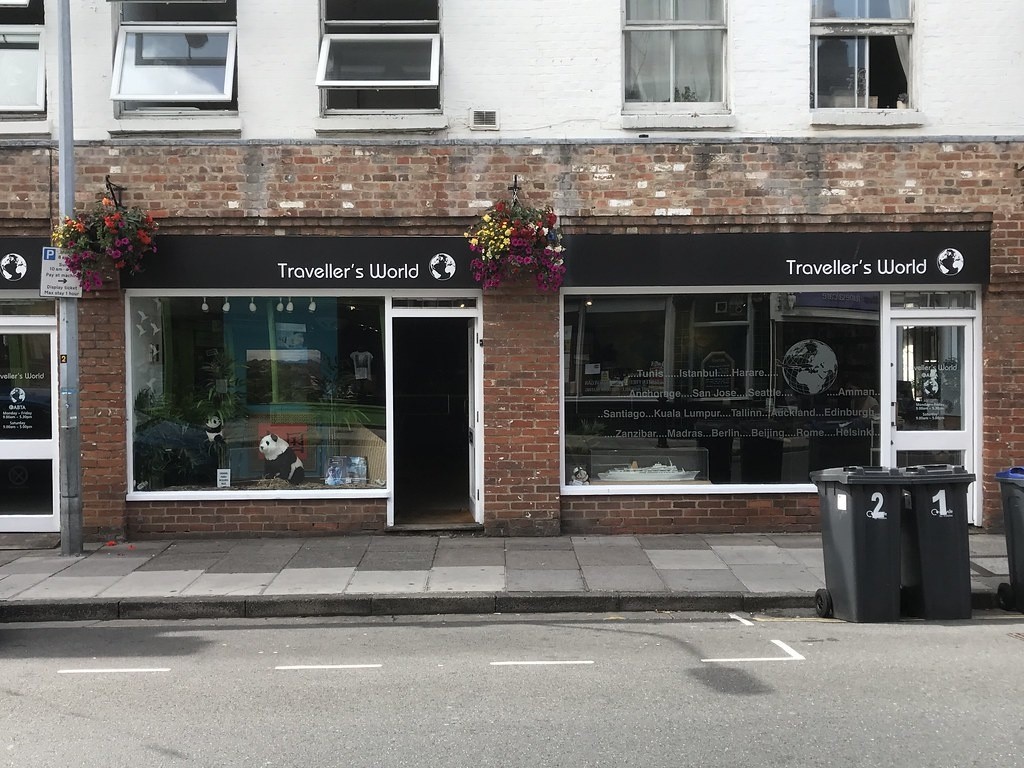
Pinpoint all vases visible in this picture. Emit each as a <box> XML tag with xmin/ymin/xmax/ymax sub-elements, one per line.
<box><xmin>84</xmin><ymin>220</ymin><xmax>121</xmax><ymax>254</ymax></box>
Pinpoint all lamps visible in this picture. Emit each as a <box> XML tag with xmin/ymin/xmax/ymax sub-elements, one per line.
<box><xmin>201</xmin><ymin>296</ymin><xmax>317</xmax><ymax>312</ymax></box>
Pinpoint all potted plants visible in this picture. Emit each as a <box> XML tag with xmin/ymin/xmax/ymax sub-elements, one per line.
<box><xmin>832</xmin><ymin>68</ymin><xmax>878</xmax><ymax>108</ymax></box>
<box><xmin>568</xmin><ymin>418</ymin><xmax>608</xmax><ymax>466</ymax></box>
<box><xmin>897</xmin><ymin>93</ymin><xmax>909</xmax><ymax>109</ymax></box>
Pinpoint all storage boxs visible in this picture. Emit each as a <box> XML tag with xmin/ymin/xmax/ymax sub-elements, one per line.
<box><xmin>324</xmin><ymin>456</ymin><xmax>367</xmax><ymax>485</ymax></box>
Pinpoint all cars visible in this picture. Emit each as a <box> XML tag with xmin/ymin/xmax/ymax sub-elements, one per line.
<box><xmin>0</xmin><ymin>387</ymin><xmax>52</xmax><ymax>503</ymax></box>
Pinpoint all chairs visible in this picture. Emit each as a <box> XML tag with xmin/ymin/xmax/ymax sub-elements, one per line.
<box><xmin>896</xmin><ymin>380</ymin><xmax>927</xmax><ymax>420</ymax></box>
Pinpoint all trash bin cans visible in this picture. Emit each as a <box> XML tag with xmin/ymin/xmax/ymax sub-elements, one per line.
<box><xmin>809</xmin><ymin>421</ymin><xmax>840</xmax><ymax>481</ymax></box>
<box><xmin>808</xmin><ymin>464</ymin><xmax>899</xmax><ymax>623</ymax></box>
<box><xmin>738</xmin><ymin>420</ymin><xmax>782</xmax><ymax>483</ymax></box>
<box><xmin>695</xmin><ymin>421</ymin><xmax>734</xmax><ymax>483</ymax></box>
<box><xmin>994</xmin><ymin>466</ymin><xmax>1024</xmax><ymax>613</ymax></box>
<box><xmin>899</xmin><ymin>464</ymin><xmax>977</xmax><ymax>621</ymax></box>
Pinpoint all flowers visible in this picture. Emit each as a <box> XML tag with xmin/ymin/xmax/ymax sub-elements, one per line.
<box><xmin>48</xmin><ymin>192</ymin><xmax>160</xmax><ymax>292</ymax></box>
<box><xmin>461</xmin><ymin>199</ymin><xmax>568</xmax><ymax>295</ymax></box>
